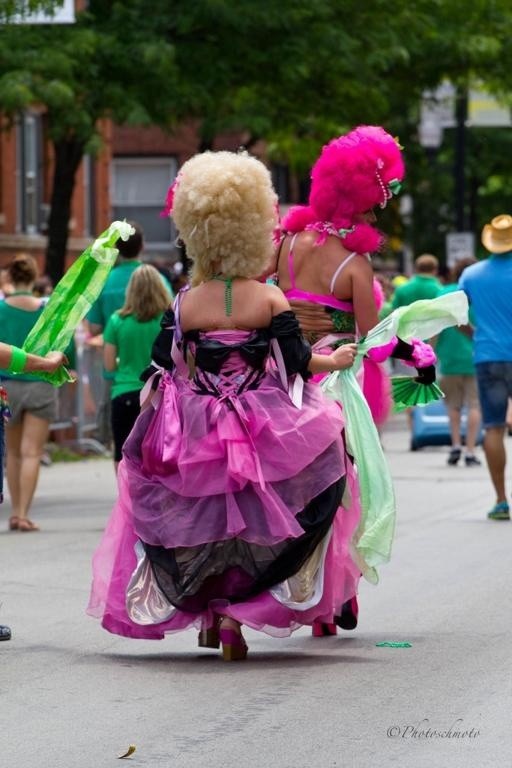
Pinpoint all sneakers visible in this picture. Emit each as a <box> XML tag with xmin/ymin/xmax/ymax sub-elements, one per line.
<box><xmin>488</xmin><ymin>499</ymin><xmax>510</xmax><ymax>520</ymax></box>
<box><xmin>448</xmin><ymin>449</ymin><xmax>460</xmax><ymax>465</ymax></box>
<box><xmin>466</xmin><ymin>456</ymin><xmax>480</xmax><ymax>467</ymax></box>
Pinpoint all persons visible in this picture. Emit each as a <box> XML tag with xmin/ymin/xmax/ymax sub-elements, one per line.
<box><xmin>263</xmin><ymin>123</ymin><xmax>439</xmax><ymax>640</ymax></box>
<box><xmin>1</xmin><ymin>249</ymin><xmax>78</xmax><ymax>531</ymax></box>
<box><xmin>85</xmin><ymin>148</ymin><xmax>359</xmax><ymax>671</ymax></box>
<box><xmin>452</xmin><ymin>213</ymin><xmax>511</xmax><ymax>522</ymax></box>
<box><xmin>82</xmin><ymin>220</ymin><xmax>176</xmax><ymax>456</ymax></box>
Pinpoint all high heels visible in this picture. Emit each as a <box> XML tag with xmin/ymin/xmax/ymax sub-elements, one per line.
<box><xmin>220</xmin><ymin>628</ymin><xmax>248</xmax><ymax>662</ymax></box>
<box><xmin>312</xmin><ymin>622</ymin><xmax>336</xmax><ymax>637</ymax></box>
<box><xmin>333</xmin><ymin>594</ymin><xmax>359</xmax><ymax>631</ymax></box>
<box><xmin>198</xmin><ymin>630</ymin><xmax>219</xmax><ymax>649</ymax></box>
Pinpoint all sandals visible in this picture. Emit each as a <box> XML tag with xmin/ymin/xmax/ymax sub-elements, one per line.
<box><xmin>9</xmin><ymin>516</ymin><xmax>37</xmax><ymax>531</ymax></box>
